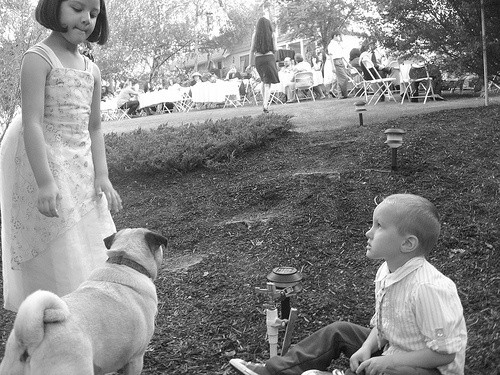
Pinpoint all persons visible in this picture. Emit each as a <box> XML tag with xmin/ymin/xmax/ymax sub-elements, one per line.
<box><xmin>115</xmin><ymin>64</ymin><xmax>261</xmax><ymax>118</ymax></box>
<box><xmin>277</xmin><ymin>51</ymin><xmax>332</xmax><ymax>102</ymax></box>
<box><xmin>349</xmin><ymin>40</ymin><xmax>394</xmax><ymax>101</ymax></box>
<box><xmin>327</xmin><ymin>31</ymin><xmax>352</xmax><ymax>99</ymax></box>
<box><xmin>0</xmin><ymin>0</ymin><xmax>123</xmax><ymax>313</ymax></box>
<box><xmin>245</xmin><ymin>17</ymin><xmax>279</xmax><ymax>113</ymax></box>
<box><xmin>229</xmin><ymin>194</ymin><xmax>466</xmax><ymax>375</ymax></box>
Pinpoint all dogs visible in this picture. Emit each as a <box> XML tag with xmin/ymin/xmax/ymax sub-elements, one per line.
<box><xmin>0</xmin><ymin>228</ymin><xmax>168</xmax><ymax>375</ymax></box>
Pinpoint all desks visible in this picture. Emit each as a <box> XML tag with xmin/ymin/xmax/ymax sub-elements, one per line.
<box><xmin>100</xmin><ymin>102</ymin><xmax>119</xmax><ymax>111</ymax></box>
<box><xmin>138</xmin><ymin>88</ymin><xmax>181</xmax><ymax>110</ymax></box>
<box><xmin>190</xmin><ymin>85</ymin><xmax>225</xmax><ymax>103</ymax></box>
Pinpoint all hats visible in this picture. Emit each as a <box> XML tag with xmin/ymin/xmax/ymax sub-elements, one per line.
<box><xmin>191</xmin><ymin>72</ymin><xmax>202</xmax><ymax>78</ymax></box>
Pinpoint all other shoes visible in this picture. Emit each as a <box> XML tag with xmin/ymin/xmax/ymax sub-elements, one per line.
<box><xmin>263</xmin><ymin>108</ymin><xmax>269</xmax><ymax>113</ymax></box>
<box><xmin>340</xmin><ymin>96</ymin><xmax>351</xmax><ymax>99</ymax></box>
<box><xmin>124</xmin><ymin>114</ymin><xmax>138</xmax><ymax>119</ymax></box>
<box><xmin>329</xmin><ymin>91</ymin><xmax>337</xmax><ymax>99</ymax></box>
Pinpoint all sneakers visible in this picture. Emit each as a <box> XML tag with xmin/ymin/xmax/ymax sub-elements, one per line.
<box><xmin>229</xmin><ymin>358</ymin><xmax>270</xmax><ymax>375</ymax></box>
<box><xmin>300</xmin><ymin>368</ymin><xmax>345</xmax><ymax>375</ymax></box>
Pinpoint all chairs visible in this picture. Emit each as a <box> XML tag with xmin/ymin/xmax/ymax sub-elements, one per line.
<box><xmin>100</xmin><ymin>57</ymin><xmax>444</xmax><ymax>122</ymax></box>
